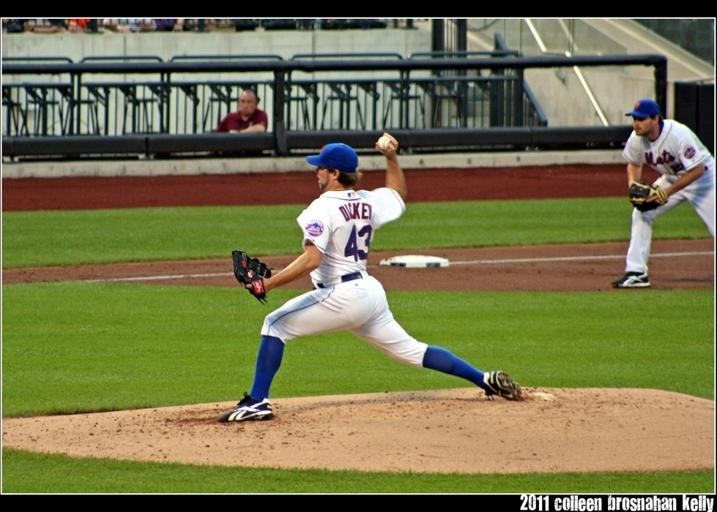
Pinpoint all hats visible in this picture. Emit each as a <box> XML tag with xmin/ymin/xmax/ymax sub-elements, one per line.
<box><xmin>625</xmin><ymin>100</ymin><xmax>660</xmax><ymax>118</ymax></box>
<box><xmin>306</xmin><ymin>143</ymin><xmax>357</xmax><ymax>172</ymax></box>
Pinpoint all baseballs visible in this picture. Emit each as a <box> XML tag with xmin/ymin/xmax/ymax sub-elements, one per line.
<box><xmin>377</xmin><ymin>137</ymin><xmax>390</xmax><ymax>150</ymax></box>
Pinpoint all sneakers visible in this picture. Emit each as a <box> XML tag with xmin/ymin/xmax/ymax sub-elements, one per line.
<box><xmin>218</xmin><ymin>393</ymin><xmax>272</xmax><ymax>422</ymax></box>
<box><xmin>612</xmin><ymin>272</ymin><xmax>650</xmax><ymax>287</ymax></box>
<box><xmin>483</xmin><ymin>370</ymin><xmax>521</xmax><ymax>400</ymax></box>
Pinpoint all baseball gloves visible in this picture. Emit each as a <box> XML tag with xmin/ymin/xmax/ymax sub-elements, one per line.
<box><xmin>232</xmin><ymin>251</ymin><xmax>271</xmax><ymax>298</ymax></box>
<box><xmin>629</xmin><ymin>179</ymin><xmax>667</xmax><ymax>211</ymax></box>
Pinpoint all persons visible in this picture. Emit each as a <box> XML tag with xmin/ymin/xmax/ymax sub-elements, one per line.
<box><xmin>207</xmin><ymin>88</ymin><xmax>268</xmax><ymax>133</ymax></box>
<box><xmin>1</xmin><ymin>19</ymin><xmax>390</xmax><ymax>31</ymax></box>
<box><xmin>608</xmin><ymin>96</ymin><xmax>715</xmax><ymax>288</ymax></box>
<box><xmin>218</xmin><ymin>132</ymin><xmax>524</xmax><ymax>421</ymax></box>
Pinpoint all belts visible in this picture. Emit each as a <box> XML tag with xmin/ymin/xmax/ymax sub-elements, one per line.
<box><xmin>317</xmin><ymin>272</ymin><xmax>362</xmax><ymax>288</ymax></box>
